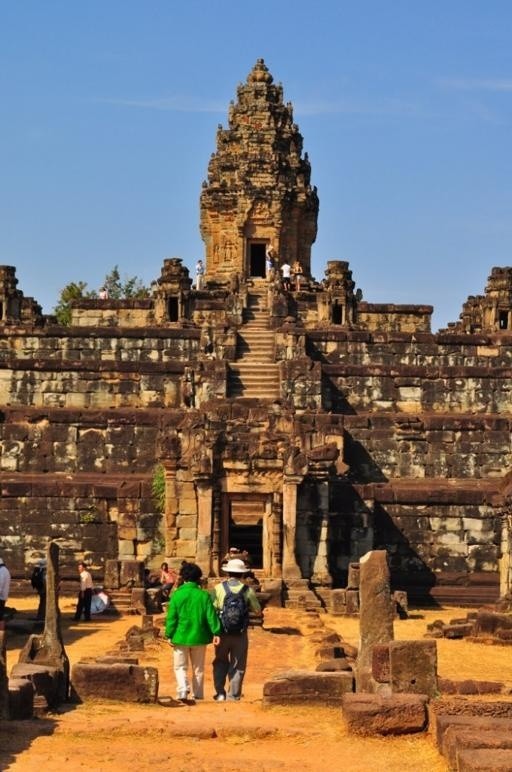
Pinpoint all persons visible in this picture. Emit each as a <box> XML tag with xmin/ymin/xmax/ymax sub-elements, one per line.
<box><xmin>195</xmin><ymin>260</ymin><xmax>204</xmax><ymax>290</ymax></box>
<box><xmin>39</xmin><ymin>560</ymin><xmax>46</xmax><ymax>621</ymax></box>
<box><xmin>265</xmin><ymin>248</ymin><xmax>274</xmax><ymax>282</ymax></box>
<box><xmin>0</xmin><ymin>558</ymin><xmax>12</xmax><ymax>632</ymax></box>
<box><xmin>280</xmin><ymin>261</ymin><xmax>291</xmax><ymax>291</ymax></box>
<box><xmin>145</xmin><ymin>547</ymin><xmax>264</xmax><ymax>701</ymax></box>
<box><xmin>71</xmin><ymin>563</ymin><xmax>93</xmax><ymax>622</ymax></box>
<box><xmin>293</xmin><ymin>263</ymin><xmax>303</xmax><ymax>291</ymax></box>
<box><xmin>99</xmin><ymin>286</ymin><xmax>109</xmax><ymax>299</ymax></box>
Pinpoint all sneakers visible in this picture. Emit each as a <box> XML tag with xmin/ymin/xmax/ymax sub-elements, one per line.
<box><xmin>176</xmin><ymin>690</ymin><xmax>188</xmax><ymax>701</ymax></box>
<box><xmin>211</xmin><ymin>691</ymin><xmax>238</xmax><ymax>702</ymax></box>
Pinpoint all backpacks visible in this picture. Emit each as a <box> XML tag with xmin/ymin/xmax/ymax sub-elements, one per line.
<box><xmin>218</xmin><ymin>580</ymin><xmax>249</xmax><ymax>635</ymax></box>
<box><xmin>30</xmin><ymin>572</ymin><xmax>43</xmax><ymax>589</ymax></box>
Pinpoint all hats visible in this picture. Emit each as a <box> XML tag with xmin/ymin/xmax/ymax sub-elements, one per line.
<box><xmin>35</xmin><ymin>558</ymin><xmax>47</xmax><ymax>569</ymax></box>
<box><xmin>220</xmin><ymin>559</ymin><xmax>250</xmax><ymax>574</ymax></box>
